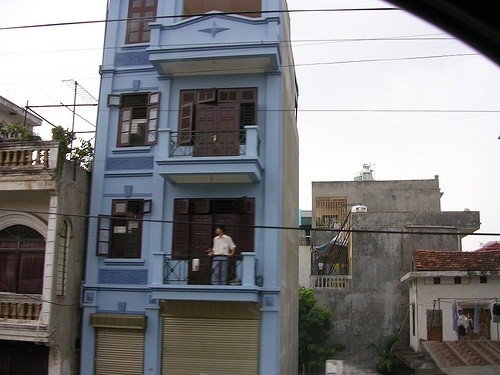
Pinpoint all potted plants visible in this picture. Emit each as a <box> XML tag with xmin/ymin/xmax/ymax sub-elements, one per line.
<box><xmin>71</xmin><ymin>139</ymin><xmax>95</xmax><ymax>164</ymax></box>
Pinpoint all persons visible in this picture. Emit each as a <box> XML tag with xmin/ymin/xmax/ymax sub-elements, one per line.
<box><xmin>456</xmin><ymin>311</ymin><xmax>475</xmax><ymax>336</ymax></box>
<box><xmin>207</xmin><ymin>224</ymin><xmax>237</xmax><ymax>287</ymax></box>
